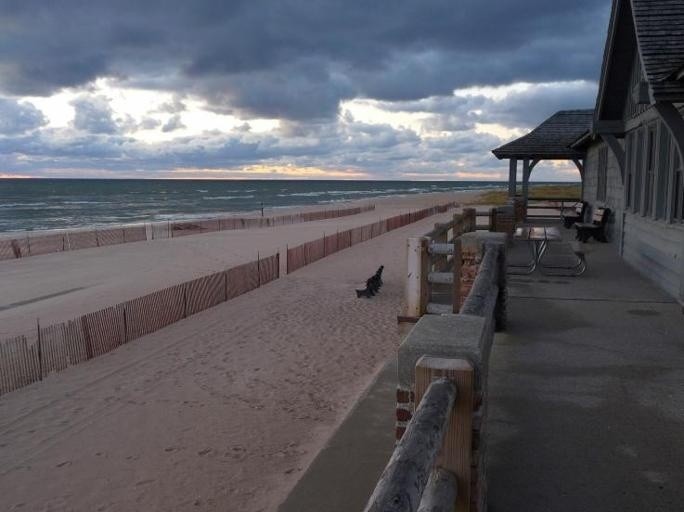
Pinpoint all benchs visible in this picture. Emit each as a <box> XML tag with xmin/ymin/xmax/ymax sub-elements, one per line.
<box><xmin>561</xmin><ymin>240</ymin><xmax>596</xmax><ymax>276</ymax></box>
<box><xmin>561</xmin><ymin>200</ymin><xmax>611</xmax><ymax>243</ymax></box>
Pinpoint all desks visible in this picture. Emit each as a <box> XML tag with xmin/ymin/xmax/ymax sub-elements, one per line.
<box><xmin>513</xmin><ymin>225</ymin><xmax>561</xmax><ymax>276</ymax></box>
